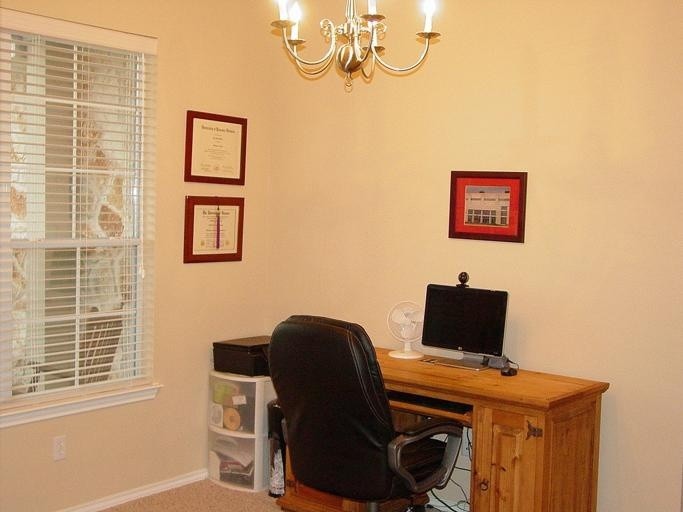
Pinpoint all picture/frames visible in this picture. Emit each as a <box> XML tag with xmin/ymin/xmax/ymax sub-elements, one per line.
<box><xmin>448</xmin><ymin>170</ymin><xmax>528</xmax><ymax>244</ymax></box>
<box><xmin>183</xmin><ymin>110</ymin><xmax>247</xmax><ymax>264</ymax></box>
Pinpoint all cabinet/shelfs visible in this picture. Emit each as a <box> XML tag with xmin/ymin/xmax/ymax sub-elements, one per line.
<box><xmin>205</xmin><ymin>373</ymin><xmax>278</xmax><ymax>493</ymax></box>
<box><xmin>275</xmin><ymin>383</ymin><xmax>601</xmax><ymax>512</ymax></box>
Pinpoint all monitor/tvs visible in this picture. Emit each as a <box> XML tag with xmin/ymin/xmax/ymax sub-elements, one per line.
<box><xmin>421</xmin><ymin>283</ymin><xmax>509</xmax><ymax>371</ymax></box>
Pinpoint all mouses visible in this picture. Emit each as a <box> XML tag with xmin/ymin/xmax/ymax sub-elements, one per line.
<box><xmin>501</xmin><ymin>367</ymin><xmax>517</xmax><ymax>376</ymax></box>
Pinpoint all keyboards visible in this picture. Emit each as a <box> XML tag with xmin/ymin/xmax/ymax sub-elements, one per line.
<box><xmin>386</xmin><ymin>389</ymin><xmax>472</xmax><ymax>415</ymax></box>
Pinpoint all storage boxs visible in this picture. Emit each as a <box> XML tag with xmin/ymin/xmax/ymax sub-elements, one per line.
<box><xmin>212</xmin><ymin>336</ymin><xmax>271</xmax><ymax>377</ymax></box>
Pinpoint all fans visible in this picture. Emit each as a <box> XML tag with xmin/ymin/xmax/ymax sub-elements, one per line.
<box><xmin>387</xmin><ymin>301</ymin><xmax>424</xmax><ymax>360</ymax></box>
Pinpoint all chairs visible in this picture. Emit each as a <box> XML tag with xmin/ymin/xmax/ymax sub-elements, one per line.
<box><xmin>266</xmin><ymin>315</ymin><xmax>463</xmax><ymax>511</ymax></box>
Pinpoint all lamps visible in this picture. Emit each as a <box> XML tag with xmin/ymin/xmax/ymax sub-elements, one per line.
<box><xmin>272</xmin><ymin>1</ymin><xmax>440</xmax><ymax>89</ymax></box>
